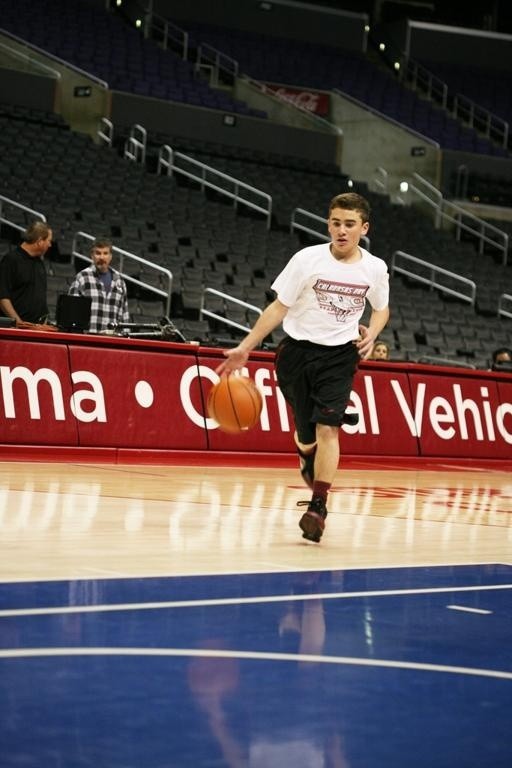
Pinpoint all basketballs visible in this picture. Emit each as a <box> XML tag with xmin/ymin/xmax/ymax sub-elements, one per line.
<box><xmin>207</xmin><ymin>376</ymin><xmax>262</xmax><ymax>435</ymax></box>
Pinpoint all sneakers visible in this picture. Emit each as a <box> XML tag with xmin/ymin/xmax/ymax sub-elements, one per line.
<box><xmin>294</xmin><ymin>430</ymin><xmax>327</xmax><ymax>542</ymax></box>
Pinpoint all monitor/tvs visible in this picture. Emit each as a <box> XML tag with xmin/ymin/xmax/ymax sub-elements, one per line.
<box><xmin>55</xmin><ymin>293</ymin><xmax>92</xmax><ymax>333</ymax></box>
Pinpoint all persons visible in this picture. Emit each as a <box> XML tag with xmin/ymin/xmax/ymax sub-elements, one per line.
<box><xmin>1</xmin><ymin>219</ymin><xmax>55</xmax><ymax>331</ymax></box>
<box><xmin>214</xmin><ymin>191</ymin><xmax>392</xmax><ymax>544</ymax></box>
<box><xmin>66</xmin><ymin>234</ymin><xmax>132</xmax><ymax>337</ymax></box>
<box><xmin>188</xmin><ymin>573</ymin><xmax>350</xmax><ymax>767</ymax></box>
<box><xmin>369</xmin><ymin>339</ymin><xmax>392</xmax><ymax>361</ymax></box>
<box><xmin>492</xmin><ymin>348</ymin><xmax>512</xmax><ymax>363</ymax></box>
